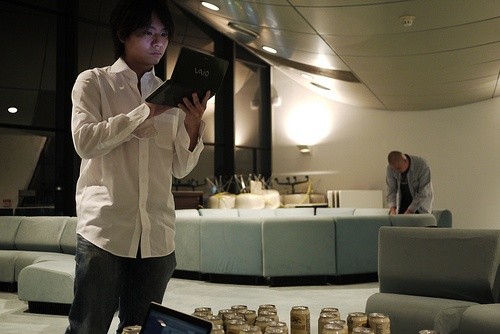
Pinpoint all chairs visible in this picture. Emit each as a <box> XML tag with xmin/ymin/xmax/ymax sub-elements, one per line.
<box><xmin>17</xmin><ymin>189</ymin><xmax>38</xmax><ymax>208</ymax></box>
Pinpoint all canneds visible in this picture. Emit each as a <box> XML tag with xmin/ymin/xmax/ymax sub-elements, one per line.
<box><xmin>122</xmin><ymin>326</ymin><xmax>141</xmax><ymax>334</ymax></box>
<box><xmin>290</xmin><ymin>306</ymin><xmax>310</xmax><ymax>334</ymax></box>
<box><xmin>318</xmin><ymin>308</ymin><xmax>391</xmax><ymax>334</ymax></box>
<box><xmin>419</xmin><ymin>329</ymin><xmax>438</xmax><ymax>334</ymax></box>
<box><xmin>192</xmin><ymin>305</ymin><xmax>289</xmax><ymax>334</ymax></box>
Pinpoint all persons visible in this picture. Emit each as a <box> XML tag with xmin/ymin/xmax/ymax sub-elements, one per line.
<box><xmin>385</xmin><ymin>151</ymin><xmax>433</xmax><ymax>215</ymax></box>
<box><xmin>65</xmin><ymin>2</ymin><xmax>210</xmax><ymax>334</ymax></box>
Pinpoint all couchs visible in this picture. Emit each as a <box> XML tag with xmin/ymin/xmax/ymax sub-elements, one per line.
<box><xmin>0</xmin><ymin>206</ymin><xmax>500</xmax><ymax>334</ymax></box>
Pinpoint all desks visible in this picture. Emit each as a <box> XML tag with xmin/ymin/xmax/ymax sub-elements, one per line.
<box><xmin>285</xmin><ymin>202</ymin><xmax>329</xmax><ymax>215</ymax></box>
<box><xmin>172</xmin><ymin>190</ymin><xmax>206</xmax><ymax>211</ymax></box>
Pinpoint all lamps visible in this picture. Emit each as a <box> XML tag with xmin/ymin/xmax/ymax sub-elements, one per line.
<box><xmin>297</xmin><ymin>146</ymin><xmax>310</xmax><ymax>153</ymax></box>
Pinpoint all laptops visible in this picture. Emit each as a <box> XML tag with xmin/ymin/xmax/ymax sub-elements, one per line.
<box><xmin>144</xmin><ymin>47</ymin><xmax>229</xmax><ymax>107</ymax></box>
<box><xmin>136</xmin><ymin>301</ymin><xmax>213</xmax><ymax>334</ymax></box>
